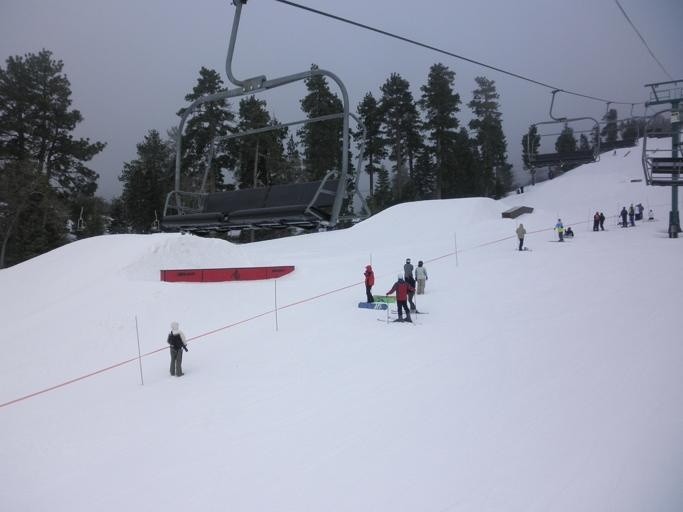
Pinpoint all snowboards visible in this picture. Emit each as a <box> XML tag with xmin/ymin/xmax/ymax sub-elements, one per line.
<box><xmin>372</xmin><ymin>296</ymin><xmax>395</xmax><ymax>303</ymax></box>
<box><xmin>358</xmin><ymin>302</ymin><xmax>388</xmax><ymax>309</ymax></box>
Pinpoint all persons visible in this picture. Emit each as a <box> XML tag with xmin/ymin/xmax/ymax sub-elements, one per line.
<box><xmin>414</xmin><ymin>261</ymin><xmax>427</xmax><ymax>294</ymax></box>
<box><xmin>592</xmin><ymin>211</ymin><xmax>600</xmax><ymax>231</ymax></box>
<box><xmin>563</xmin><ymin>227</ymin><xmax>574</xmax><ymax>237</ymax></box>
<box><xmin>515</xmin><ymin>224</ymin><xmax>526</xmax><ymax>251</ymax></box>
<box><xmin>402</xmin><ymin>258</ymin><xmax>413</xmax><ymax>280</ymax></box>
<box><xmin>166</xmin><ymin>321</ymin><xmax>187</xmax><ymax>376</ymax></box>
<box><xmin>363</xmin><ymin>264</ymin><xmax>374</xmax><ymax>303</ymax></box>
<box><xmin>618</xmin><ymin>203</ymin><xmax>655</xmax><ymax>228</ymax></box>
<box><xmin>553</xmin><ymin>219</ymin><xmax>564</xmax><ymax>241</ymax></box>
<box><xmin>599</xmin><ymin>212</ymin><xmax>605</xmax><ymax>230</ymax></box>
<box><xmin>385</xmin><ymin>275</ymin><xmax>416</xmax><ymax>323</ymax></box>
<box><xmin>406</xmin><ymin>275</ymin><xmax>415</xmax><ymax>310</ymax></box>
